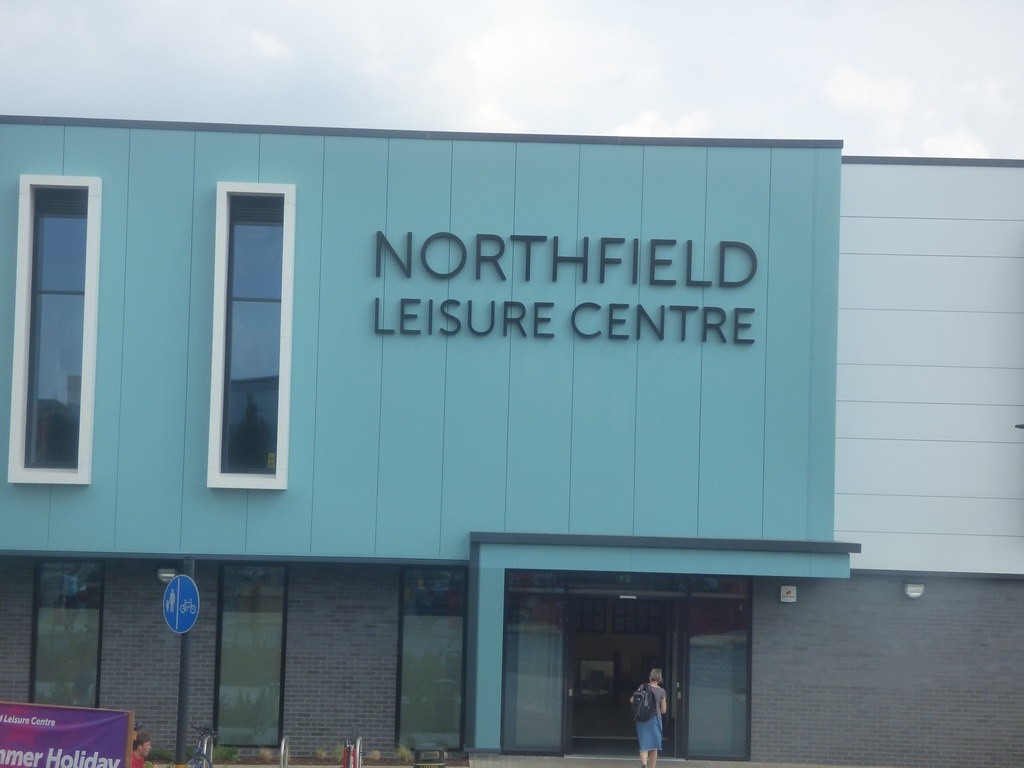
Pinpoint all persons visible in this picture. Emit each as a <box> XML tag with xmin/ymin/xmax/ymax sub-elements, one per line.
<box><xmin>630</xmin><ymin>669</ymin><xmax>666</xmax><ymax>768</ymax></box>
<box><xmin>132</xmin><ymin>733</ymin><xmax>151</xmax><ymax>768</ymax></box>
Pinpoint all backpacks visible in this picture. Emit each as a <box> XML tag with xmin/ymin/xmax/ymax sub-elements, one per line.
<box><xmin>631</xmin><ymin>683</ymin><xmax>657</xmax><ymax>722</ymax></box>
